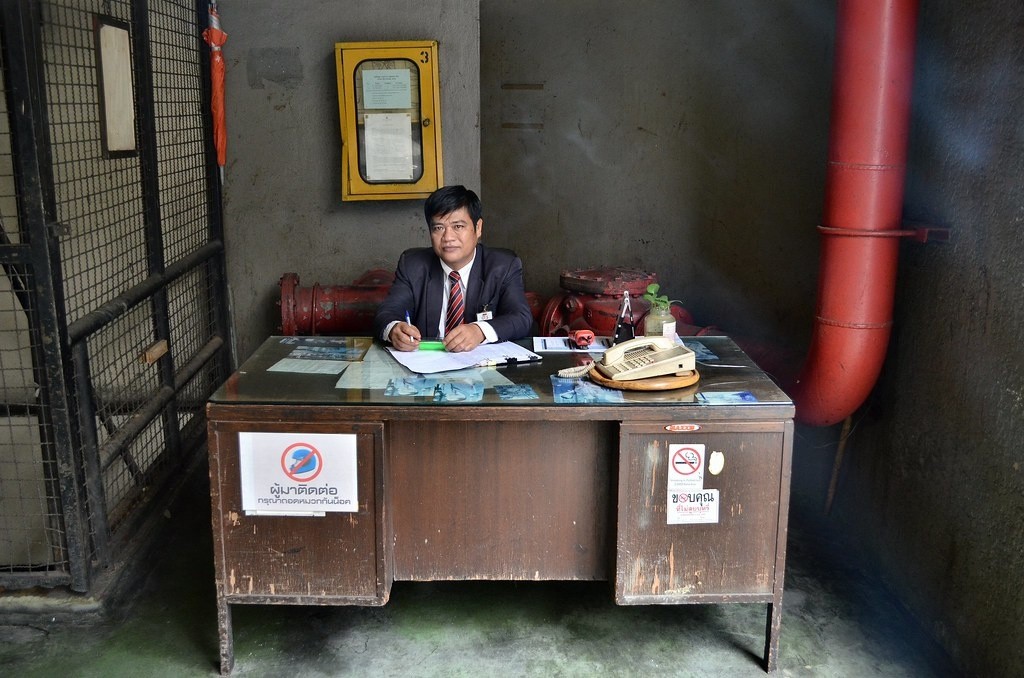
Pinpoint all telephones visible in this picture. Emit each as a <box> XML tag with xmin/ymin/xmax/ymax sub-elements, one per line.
<box><xmin>595</xmin><ymin>336</ymin><xmax>695</xmax><ymax>380</ymax></box>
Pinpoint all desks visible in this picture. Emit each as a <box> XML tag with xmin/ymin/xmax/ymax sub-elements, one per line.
<box><xmin>205</xmin><ymin>334</ymin><xmax>795</xmax><ymax>677</ymax></box>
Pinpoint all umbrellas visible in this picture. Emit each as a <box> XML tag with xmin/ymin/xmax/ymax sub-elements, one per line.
<box><xmin>202</xmin><ymin>0</ymin><xmax>229</xmax><ymax>185</ymax></box>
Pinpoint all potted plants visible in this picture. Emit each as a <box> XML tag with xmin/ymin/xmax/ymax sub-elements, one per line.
<box><xmin>644</xmin><ymin>283</ymin><xmax>683</xmax><ymax>342</ymax></box>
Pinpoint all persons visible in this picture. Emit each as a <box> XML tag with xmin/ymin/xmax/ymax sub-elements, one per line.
<box><xmin>374</xmin><ymin>186</ymin><xmax>533</xmax><ymax>353</ymax></box>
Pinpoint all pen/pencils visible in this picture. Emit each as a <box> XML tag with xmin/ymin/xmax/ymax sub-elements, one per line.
<box><xmin>405</xmin><ymin>310</ymin><xmax>414</xmax><ymax>343</ymax></box>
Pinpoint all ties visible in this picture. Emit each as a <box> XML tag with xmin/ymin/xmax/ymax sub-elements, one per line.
<box><xmin>445</xmin><ymin>270</ymin><xmax>464</xmax><ymax>339</ymax></box>
